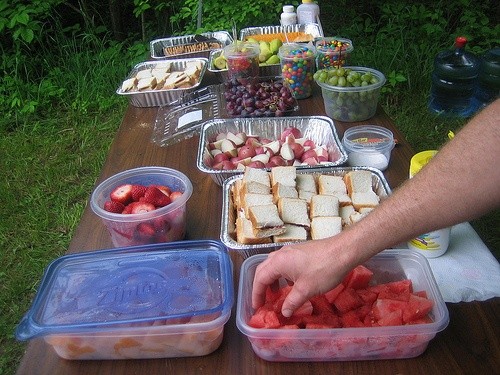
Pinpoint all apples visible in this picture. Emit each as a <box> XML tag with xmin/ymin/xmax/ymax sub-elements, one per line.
<box><xmin>203</xmin><ymin>126</ymin><xmax>329</xmax><ymax>185</ymax></box>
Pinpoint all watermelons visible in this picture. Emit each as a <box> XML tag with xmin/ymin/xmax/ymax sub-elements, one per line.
<box><xmin>251</xmin><ymin>264</ymin><xmax>434</xmax><ymax>360</ymax></box>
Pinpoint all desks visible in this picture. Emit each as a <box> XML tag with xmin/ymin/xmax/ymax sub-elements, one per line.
<box><xmin>15</xmin><ymin>78</ymin><xmax>500</xmax><ymax>375</ymax></box>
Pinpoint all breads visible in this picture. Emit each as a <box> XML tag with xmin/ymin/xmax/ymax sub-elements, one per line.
<box><xmin>232</xmin><ymin>166</ymin><xmax>381</xmax><ymax>245</ymax></box>
<box><xmin>122</xmin><ymin>62</ymin><xmax>202</xmax><ymax>92</ymax></box>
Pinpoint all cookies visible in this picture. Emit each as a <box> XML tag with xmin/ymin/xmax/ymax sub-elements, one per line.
<box><xmin>162</xmin><ymin>42</ymin><xmax>219</xmax><ymax>56</ymax></box>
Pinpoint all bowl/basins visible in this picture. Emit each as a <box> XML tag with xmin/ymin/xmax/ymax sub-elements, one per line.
<box><xmin>14</xmin><ymin>239</ymin><xmax>235</xmax><ymax>360</ymax></box>
<box><xmin>342</xmin><ymin>125</ymin><xmax>395</xmax><ymax>171</ymax></box>
<box><xmin>316</xmin><ymin>66</ymin><xmax>387</xmax><ymax>122</ymax></box>
<box><xmin>235</xmin><ymin>251</ymin><xmax>451</xmax><ymax>362</ymax></box>
<box><xmin>90</xmin><ymin>166</ymin><xmax>193</xmax><ymax>248</ymax></box>
<box><xmin>152</xmin><ymin>74</ymin><xmax>300</xmax><ymax>149</ymax></box>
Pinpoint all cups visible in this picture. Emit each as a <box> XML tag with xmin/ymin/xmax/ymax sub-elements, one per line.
<box><xmin>277</xmin><ymin>43</ymin><xmax>318</xmax><ymax>99</ymax></box>
<box><xmin>315</xmin><ymin>37</ymin><xmax>354</xmax><ymax>71</ymax></box>
<box><xmin>223</xmin><ymin>42</ymin><xmax>261</xmax><ymax>81</ymax></box>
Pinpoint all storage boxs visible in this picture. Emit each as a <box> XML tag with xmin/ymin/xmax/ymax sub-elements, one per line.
<box><xmin>235</xmin><ymin>250</ymin><xmax>450</xmax><ymax>362</ymax></box>
<box><xmin>115</xmin><ymin>23</ymin><xmax>324</xmax><ymax>147</ymax></box>
<box><xmin>16</xmin><ymin>238</ymin><xmax>233</xmax><ymax>362</ymax></box>
<box><xmin>314</xmin><ymin>67</ymin><xmax>387</xmax><ymax>122</ymax></box>
<box><xmin>198</xmin><ymin>114</ymin><xmax>394</xmax><ymax>257</ymax></box>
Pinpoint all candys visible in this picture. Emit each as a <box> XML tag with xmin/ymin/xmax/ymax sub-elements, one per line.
<box><xmin>283</xmin><ymin>39</ymin><xmax>350</xmax><ymax>98</ymax></box>
<box><xmin>228</xmin><ymin>54</ymin><xmax>257</xmax><ymax>83</ymax></box>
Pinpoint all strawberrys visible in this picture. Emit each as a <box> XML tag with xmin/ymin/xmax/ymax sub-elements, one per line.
<box><xmin>104</xmin><ymin>185</ymin><xmax>186</xmax><ymax>248</ymax></box>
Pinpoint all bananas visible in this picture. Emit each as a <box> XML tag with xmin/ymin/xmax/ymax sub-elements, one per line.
<box><xmin>215</xmin><ymin>37</ymin><xmax>282</xmax><ymax>71</ymax></box>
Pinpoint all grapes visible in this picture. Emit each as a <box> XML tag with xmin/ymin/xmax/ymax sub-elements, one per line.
<box><xmin>224</xmin><ymin>77</ymin><xmax>295</xmax><ymax>118</ymax></box>
<box><xmin>313</xmin><ymin>65</ymin><xmax>378</xmax><ymax>120</ymax></box>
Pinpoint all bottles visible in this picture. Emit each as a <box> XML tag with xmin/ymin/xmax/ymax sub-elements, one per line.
<box><xmin>480</xmin><ymin>43</ymin><xmax>500</xmax><ymax>98</ymax></box>
<box><xmin>280</xmin><ymin>5</ymin><xmax>297</xmax><ymax>26</ymax></box>
<box><xmin>297</xmin><ymin>0</ymin><xmax>320</xmax><ymax>24</ymax></box>
<box><xmin>408</xmin><ymin>150</ymin><xmax>452</xmax><ymax>259</ymax></box>
<box><xmin>429</xmin><ymin>35</ymin><xmax>484</xmax><ymax>117</ymax></box>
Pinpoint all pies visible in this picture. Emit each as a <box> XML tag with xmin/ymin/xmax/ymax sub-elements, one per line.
<box><xmin>246</xmin><ymin>32</ymin><xmax>313</xmax><ymax>42</ymax></box>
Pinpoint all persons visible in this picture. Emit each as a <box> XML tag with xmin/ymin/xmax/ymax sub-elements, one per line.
<box><xmin>251</xmin><ymin>98</ymin><xmax>499</xmax><ymax>317</ymax></box>
